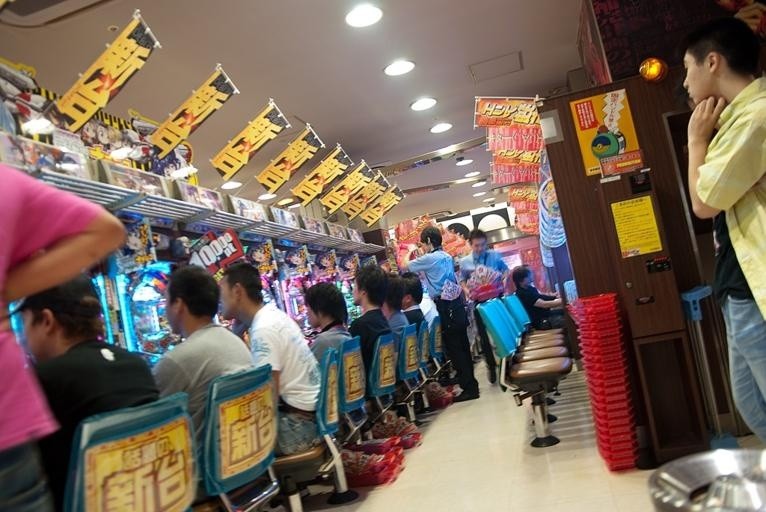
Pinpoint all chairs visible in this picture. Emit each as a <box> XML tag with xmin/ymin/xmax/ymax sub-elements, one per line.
<box><xmin>475</xmin><ymin>292</ymin><xmax>576</xmax><ymax>451</ymax></box>
<box><xmin>337</xmin><ymin>317</ymin><xmax>452</xmax><ymax>453</ymax></box>
<box><xmin>61</xmin><ymin>392</ymin><xmax>198</xmax><ymax>512</ymax></box>
<box><xmin>194</xmin><ymin>364</ymin><xmax>280</xmax><ymax>512</ymax></box>
<box><xmin>276</xmin><ymin>348</ymin><xmax>359</xmax><ymax>512</ymax></box>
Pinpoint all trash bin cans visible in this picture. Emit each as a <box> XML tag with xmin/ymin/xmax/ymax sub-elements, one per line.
<box><xmin>648</xmin><ymin>448</ymin><xmax>766</xmax><ymax>512</ymax></box>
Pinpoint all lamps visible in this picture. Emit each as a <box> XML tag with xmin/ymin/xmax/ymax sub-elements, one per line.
<box><xmin>637</xmin><ymin>55</ymin><xmax>685</xmax><ymax>84</ymax></box>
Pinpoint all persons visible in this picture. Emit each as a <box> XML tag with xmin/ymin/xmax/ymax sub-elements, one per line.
<box><xmin>683</xmin><ymin>15</ymin><xmax>765</xmax><ymax>445</ymax></box>
<box><xmin>380</xmin><ymin>271</ymin><xmax>410</xmax><ymax>353</ymax></box>
<box><xmin>0</xmin><ymin>163</ymin><xmax>126</xmax><ymax>512</ymax></box>
<box><xmin>459</xmin><ymin>229</ymin><xmax>509</xmax><ymax>385</ymax></box>
<box><xmin>400</xmin><ymin>271</ymin><xmax>426</xmax><ymax>337</ymax></box>
<box><xmin>151</xmin><ymin>265</ymin><xmax>256</xmax><ymax>503</ymax></box>
<box><xmin>348</xmin><ymin>266</ymin><xmax>393</xmax><ymax>422</ymax></box>
<box><xmin>219</xmin><ymin>263</ymin><xmax>324</xmax><ymax>458</ymax></box>
<box><xmin>512</xmin><ymin>268</ymin><xmax>567</xmax><ymax>330</ymax></box>
<box><xmin>9</xmin><ymin>276</ymin><xmax>160</xmax><ymax>509</ymax></box>
<box><xmin>306</xmin><ymin>282</ymin><xmax>352</xmax><ymax>364</ymax></box>
<box><xmin>400</xmin><ymin>227</ymin><xmax>480</xmax><ymax>402</ymax></box>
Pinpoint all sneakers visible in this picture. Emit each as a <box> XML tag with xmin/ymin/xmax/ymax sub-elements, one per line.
<box><xmin>452</xmin><ymin>391</ymin><xmax>480</xmax><ymax>402</ymax></box>
<box><xmin>487</xmin><ymin>366</ymin><xmax>496</xmax><ymax>384</ymax></box>
